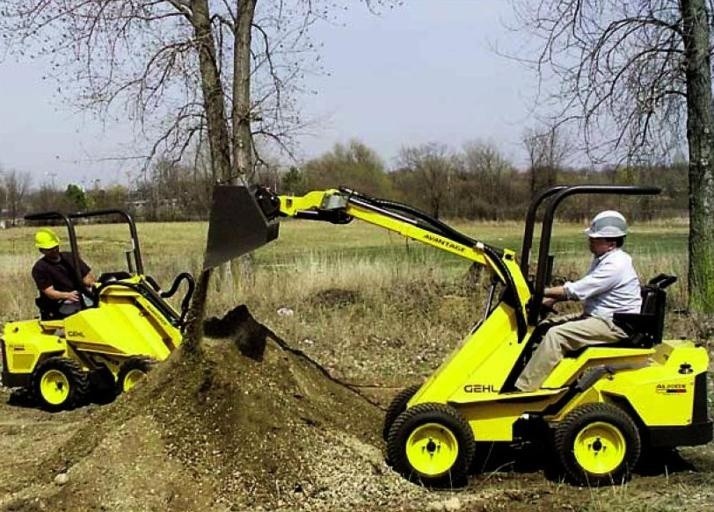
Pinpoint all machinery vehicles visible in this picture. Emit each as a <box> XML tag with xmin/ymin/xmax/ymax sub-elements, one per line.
<box><xmin>1</xmin><ymin>209</ymin><xmax>194</xmax><ymax>409</ymax></box>
<box><xmin>201</xmin><ymin>185</ymin><xmax>713</xmax><ymax>489</ymax></box>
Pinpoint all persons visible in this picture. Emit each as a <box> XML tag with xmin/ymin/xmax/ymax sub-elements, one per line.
<box><xmin>29</xmin><ymin>225</ymin><xmax>97</xmax><ymax>321</ymax></box>
<box><xmin>510</xmin><ymin>210</ymin><xmax>644</xmax><ymax>394</ymax></box>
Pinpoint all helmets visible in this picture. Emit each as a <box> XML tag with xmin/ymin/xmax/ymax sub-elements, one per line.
<box><xmin>584</xmin><ymin>209</ymin><xmax>627</xmax><ymax>238</ymax></box>
<box><xmin>34</xmin><ymin>227</ymin><xmax>60</xmax><ymax>250</ymax></box>
